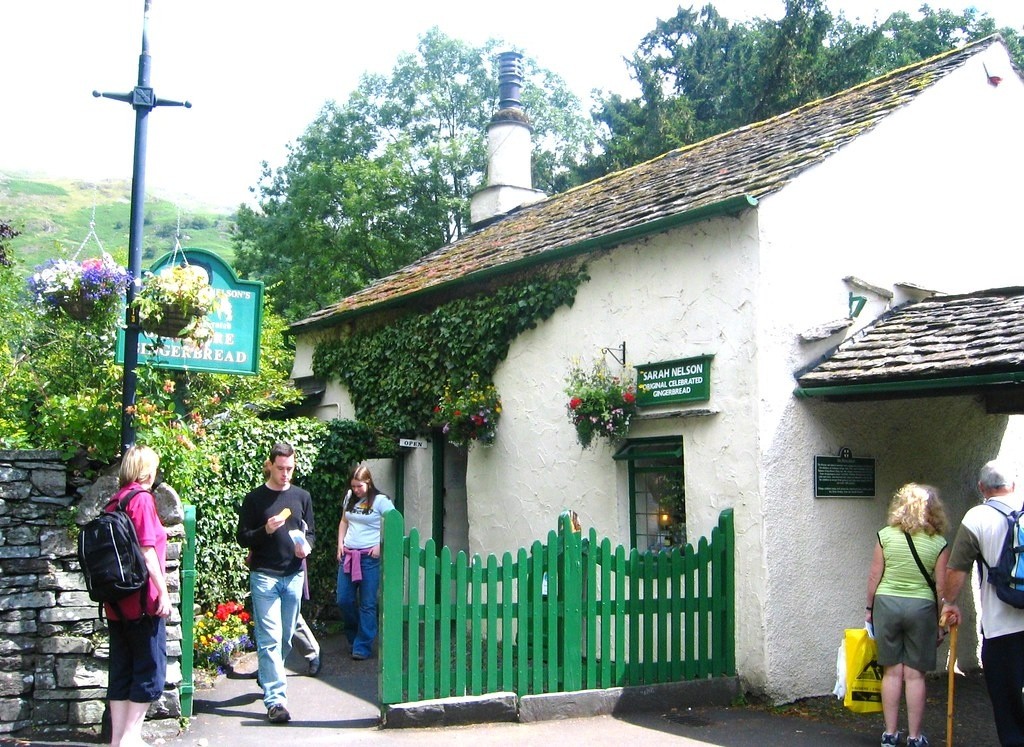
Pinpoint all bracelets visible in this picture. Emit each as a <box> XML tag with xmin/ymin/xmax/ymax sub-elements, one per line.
<box><xmin>941</xmin><ymin>598</ymin><xmax>955</xmax><ymax>606</ymax></box>
<box><xmin>865</xmin><ymin>606</ymin><xmax>872</xmax><ymax>610</ymax></box>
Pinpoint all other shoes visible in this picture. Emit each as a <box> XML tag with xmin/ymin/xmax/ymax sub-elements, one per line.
<box><xmin>352</xmin><ymin>653</ymin><xmax>368</xmax><ymax>659</ymax></box>
<box><xmin>308</xmin><ymin>647</ymin><xmax>321</xmax><ymax>676</ymax></box>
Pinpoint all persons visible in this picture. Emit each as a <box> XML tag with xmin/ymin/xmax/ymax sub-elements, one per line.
<box><xmin>862</xmin><ymin>482</ymin><xmax>952</xmax><ymax>747</ymax></box>
<box><xmin>336</xmin><ymin>464</ymin><xmax>395</xmax><ymax>659</ymax></box>
<box><xmin>235</xmin><ymin>443</ymin><xmax>318</xmax><ymax>723</ymax></box>
<box><xmin>102</xmin><ymin>442</ymin><xmax>173</xmax><ymax>747</ymax></box>
<box><xmin>940</xmin><ymin>459</ymin><xmax>1024</xmax><ymax>747</ymax></box>
<box><xmin>245</xmin><ymin>548</ymin><xmax>323</xmax><ymax>677</ymax></box>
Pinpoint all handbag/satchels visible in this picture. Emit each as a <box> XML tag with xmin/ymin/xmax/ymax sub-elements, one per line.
<box><xmin>832</xmin><ymin>621</ymin><xmax>884</xmax><ymax>713</ymax></box>
<box><xmin>936</xmin><ymin>622</ymin><xmax>946</xmax><ymax>647</ymax></box>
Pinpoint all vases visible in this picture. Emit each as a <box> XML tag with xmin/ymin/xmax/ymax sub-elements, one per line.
<box><xmin>60</xmin><ymin>298</ymin><xmax>95</xmax><ymax>319</ymax></box>
<box><xmin>147</xmin><ymin>303</ymin><xmax>204</xmax><ymax>340</ymax></box>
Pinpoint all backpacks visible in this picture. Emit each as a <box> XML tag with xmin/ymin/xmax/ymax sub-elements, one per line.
<box><xmin>77</xmin><ymin>490</ymin><xmax>152</xmax><ymax>603</ymax></box>
<box><xmin>977</xmin><ymin>501</ymin><xmax>1024</xmax><ymax>610</ymax></box>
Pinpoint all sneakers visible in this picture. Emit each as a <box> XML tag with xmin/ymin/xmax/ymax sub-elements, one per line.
<box><xmin>268</xmin><ymin>703</ymin><xmax>291</xmax><ymax>723</ymax></box>
<box><xmin>880</xmin><ymin>729</ymin><xmax>903</xmax><ymax>747</ymax></box>
<box><xmin>906</xmin><ymin>734</ymin><xmax>929</xmax><ymax>747</ymax></box>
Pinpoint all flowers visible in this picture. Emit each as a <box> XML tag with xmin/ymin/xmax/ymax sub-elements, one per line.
<box><xmin>424</xmin><ymin>371</ymin><xmax>503</xmax><ymax>448</ymax></box>
<box><xmin>30</xmin><ymin>251</ymin><xmax>129</xmax><ymax>339</ymax></box>
<box><xmin>564</xmin><ymin>346</ymin><xmax>646</xmax><ymax>451</ymax></box>
<box><xmin>128</xmin><ymin>265</ymin><xmax>223</xmax><ymax>366</ymax></box>
